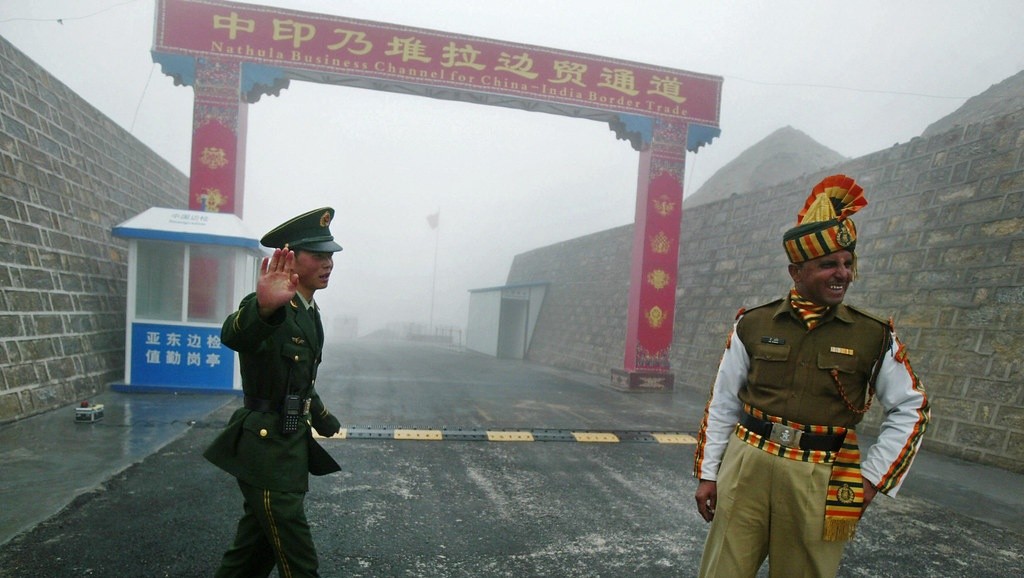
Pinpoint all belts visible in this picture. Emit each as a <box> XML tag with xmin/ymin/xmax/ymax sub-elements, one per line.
<box><xmin>244</xmin><ymin>396</ymin><xmax>311</xmax><ymax>416</ymax></box>
<box><xmin>739</xmin><ymin>409</ymin><xmax>847</xmax><ymax>451</ymax></box>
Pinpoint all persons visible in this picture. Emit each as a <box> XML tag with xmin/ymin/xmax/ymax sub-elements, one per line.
<box><xmin>205</xmin><ymin>206</ymin><xmax>342</xmax><ymax>578</ymax></box>
<box><xmin>693</xmin><ymin>216</ymin><xmax>930</xmax><ymax>578</ymax></box>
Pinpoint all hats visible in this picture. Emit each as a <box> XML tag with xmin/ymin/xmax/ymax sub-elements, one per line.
<box><xmin>783</xmin><ymin>175</ymin><xmax>868</xmax><ymax>287</ymax></box>
<box><xmin>260</xmin><ymin>206</ymin><xmax>343</xmax><ymax>252</ymax></box>
